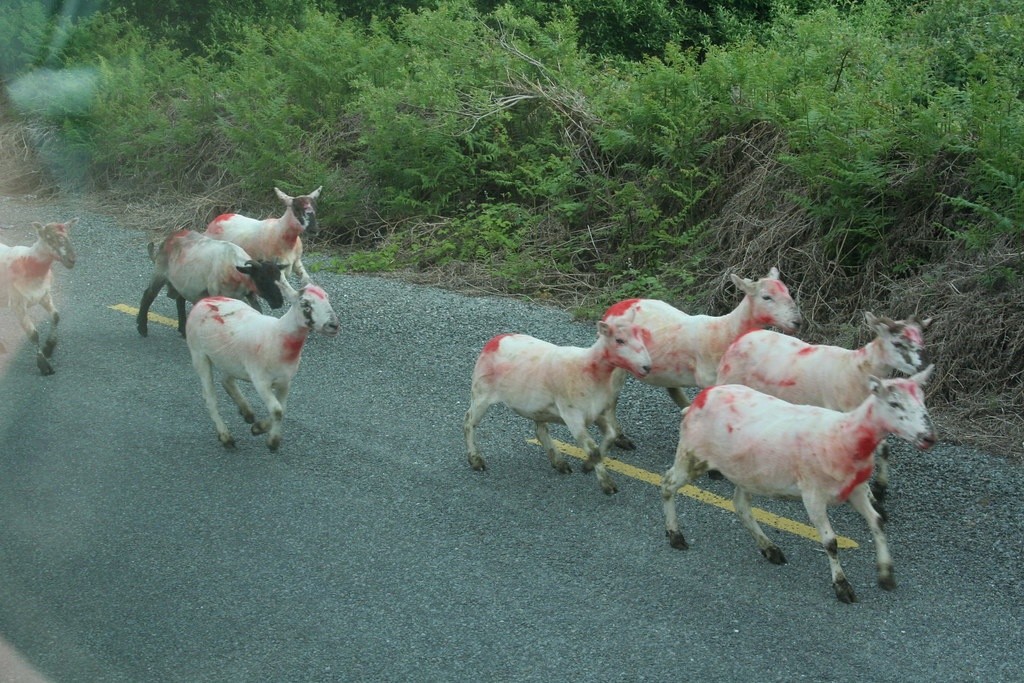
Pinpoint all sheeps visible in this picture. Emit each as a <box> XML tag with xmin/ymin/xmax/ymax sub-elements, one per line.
<box><xmin>135</xmin><ymin>228</ymin><xmax>290</xmax><ymax>340</ymax></box>
<box><xmin>595</xmin><ymin>266</ymin><xmax>803</xmax><ymax>453</ymax></box>
<box><xmin>203</xmin><ymin>185</ymin><xmax>324</xmax><ymax>303</ymax></box>
<box><xmin>461</xmin><ymin>308</ymin><xmax>654</xmax><ymax>496</ymax></box>
<box><xmin>706</xmin><ymin>310</ymin><xmax>936</xmax><ymax>504</ymax></box>
<box><xmin>0</xmin><ymin>217</ymin><xmax>79</xmax><ymax>377</ymax></box>
<box><xmin>661</xmin><ymin>363</ymin><xmax>937</xmax><ymax>605</ymax></box>
<box><xmin>185</xmin><ymin>272</ymin><xmax>340</xmax><ymax>453</ymax></box>
